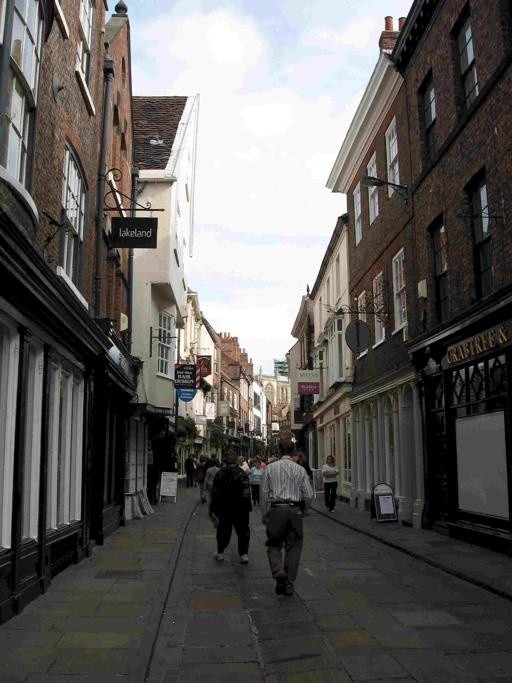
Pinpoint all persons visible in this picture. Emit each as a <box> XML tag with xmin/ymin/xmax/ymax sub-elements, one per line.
<box><xmin>184</xmin><ymin>450</ymin><xmax>342</xmax><ymax>565</ymax></box>
<box><xmin>258</xmin><ymin>438</ymin><xmax>316</xmax><ymax>596</ymax></box>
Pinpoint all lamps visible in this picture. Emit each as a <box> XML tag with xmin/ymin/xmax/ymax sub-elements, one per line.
<box><xmin>359</xmin><ymin>173</ymin><xmax>410</xmax><ymax>207</ymax></box>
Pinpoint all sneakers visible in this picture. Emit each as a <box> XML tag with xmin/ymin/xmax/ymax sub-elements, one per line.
<box><xmin>215</xmin><ymin>553</ymin><xmax>224</xmax><ymax>560</ymax></box>
<box><xmin>240</xmin><ymin>555</ymin><xmax>249</xmax><ymax>563</ymax></box>
<box><xmin>276</xmin><ymin>576</ymin><xmax>294</xmax><ymax>596</ymax></box>
